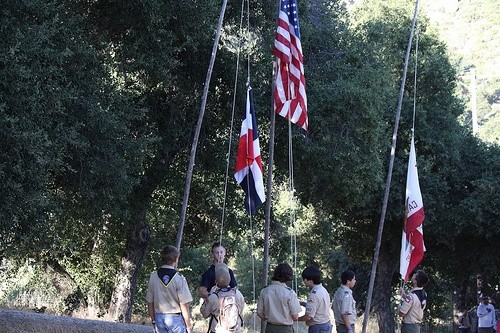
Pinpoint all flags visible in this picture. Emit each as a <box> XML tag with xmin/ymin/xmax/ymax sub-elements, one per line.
<box><xmin>271</xmin><ymin>0</ymin><xmax>309</xmax><ymax>133</ymax></box>
<box><xmin>398</xmin><ymin>127</ymin><xmax>426</xmax><ymax>283</ymax></box>
<box><xmin>234</xmin><ymin>76</ymin><xmax>266</xmax><ymax>217</ymax></box>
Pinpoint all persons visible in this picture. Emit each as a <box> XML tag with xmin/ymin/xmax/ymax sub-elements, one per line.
<box><xmin>478</xmin><ymin>297</ymin><xmax>496</xmax><ymax>332</ymax></box>
<box><xmin>145</xmin><ymin>245</ymin><xmax>193</xmax><ymax>333</ymax></box>
<box><xmin>200</xmin><ymin>263</ymin><xmax>250</xmax><ymax>333</ymax></box>
<box><xmin>294</xmin><ymin>265</ymin><xmax>333</xmax><ymax>333</ymax></box>
<box><xmin>331</xmin><ymin>270</ymin><xmax>357</xmax><ymax>333</ymax></box>
<box><xmin>399</xmin><ymin>270</ymin><xmax>428</xmax><ymax>333</ymax></box>
<box><xmin>257</xmin><ymin>263</ymin><xmax>302</xmax><ymax>333</ymax></box>
<box><xmin>198</xmin><ymin>241</ymin><xmax>237</xmax><ymax>298</ymax></box>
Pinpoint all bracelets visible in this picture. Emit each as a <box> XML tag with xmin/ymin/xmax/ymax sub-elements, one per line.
<box><xmin>151</xmin><ymin>320</ymin><xmax>156</xmax><ymax>324</ymax></box>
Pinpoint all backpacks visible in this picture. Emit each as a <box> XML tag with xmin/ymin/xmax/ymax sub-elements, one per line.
<box><xmin>212</xmin><ymin>288</ymin><xmax>240</xmax><ymax>331</ymax></box>
